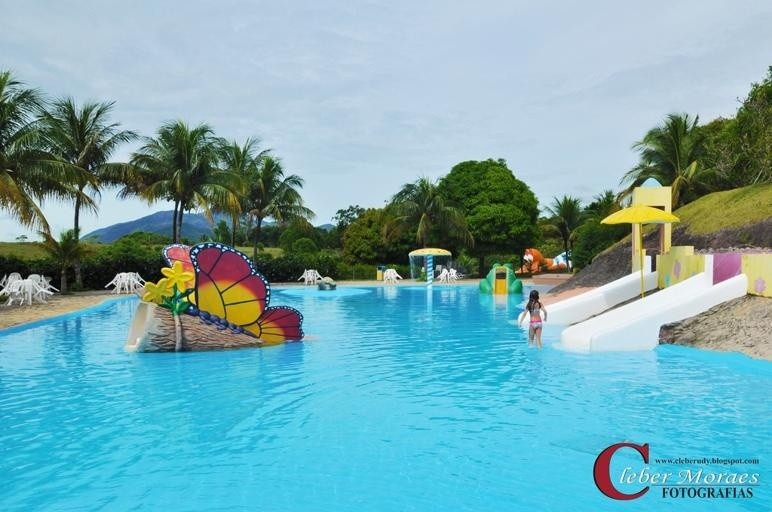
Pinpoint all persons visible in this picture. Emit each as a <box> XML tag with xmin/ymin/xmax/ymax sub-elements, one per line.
<box><xmin>520</xmin><ymin>290</ymin><xmax>547</xmax><ymax>349</ymax></box>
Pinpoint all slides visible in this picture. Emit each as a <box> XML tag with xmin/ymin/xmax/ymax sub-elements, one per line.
<box><xmin>480</xmin><ymin>262</ymin><xmax>523</xmax><ymax>294</ymax></box>
<box><xmin>562</xmin><ymin>272</ymin><xmax>748</xmax><ymax>353</ymax></box>
<box><xmin>517</xmin><ymin>271</ymin><xmax>661</xmax><ymax>331</ymax></box>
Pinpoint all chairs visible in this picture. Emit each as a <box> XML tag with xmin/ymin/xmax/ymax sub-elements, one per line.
<box><xmin>384</xmin><ymin>268</ymin><xmax>403</xmax><ymax>285</ymax></box>
<box><xmin>104</xmin><ymin>272</ymin><xmax>146</xmax><ymax>294</ymax></box>
<box><xmin>1</xmin><ymin>272</ymin><xmax>59</xmax><ymax>306</ymax></box>
<box><xmin>298</xmin><ymin>269</ymin><xmax>323</xmax><ymax>285</ymax></box>
<box><xmin>437</xmin><ymin>268</ymin><xmax>460</xmax><ymax>285</ymax></box>
